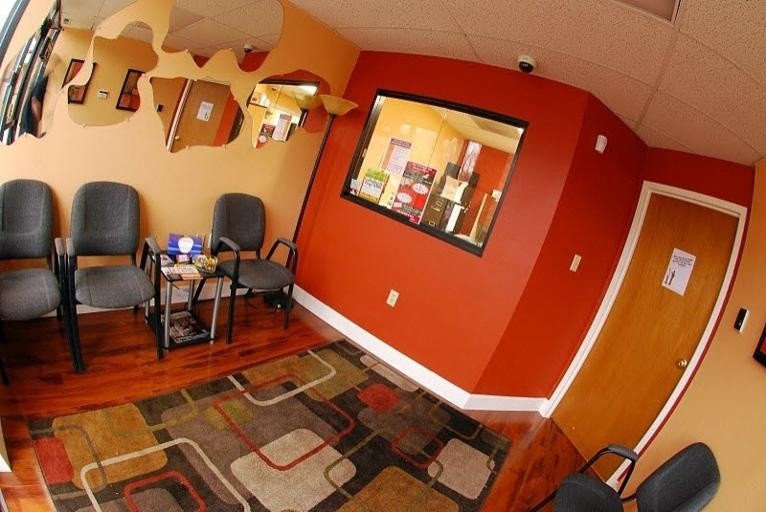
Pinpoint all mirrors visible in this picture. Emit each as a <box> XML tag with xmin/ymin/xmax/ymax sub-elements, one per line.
<box><xmin>161</xmin><ymin>0</ymin><xmax>284</xmax><ymax>73</ymax></box>
<box><xmin>148</xmin><ymin>76</ymin><xmax>245</xmax><ymax>153</ymax></box>
<box><xmin>67</xmin><ymin>19</ymin><xmax>159</xmax><ymax>127</ymax></box>
<box><xmin>244</xmin><ymin>68</ymin><xmax>330</xmax><ymax>149</ymax></box>
<box><xmin>1</xmin><ymin>1</ymin><xmax>65</xmax><ymax>145</ymax></box>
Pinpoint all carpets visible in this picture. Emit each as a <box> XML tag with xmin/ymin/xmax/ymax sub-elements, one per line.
<box><xmin>0</xmin><ymin>337</ymin><xmax>512</xmax><ymax>512</ymax></box>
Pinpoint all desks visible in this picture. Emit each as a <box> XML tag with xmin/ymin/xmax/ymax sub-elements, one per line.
<box><xmin>158</xmin><ymin>251</ymin><xmax>226</xmax><ymax>351</ymax></box>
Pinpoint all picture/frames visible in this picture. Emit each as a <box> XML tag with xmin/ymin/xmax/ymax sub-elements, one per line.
<box><xmin>752</xmin><ymin>323</ymin><xmax>766</xmax><ymax>370</ymax></box>
<box><xmin>116</xmin><ymin>69</ymin><xmax>147</xmax><ymax>111</ymax></box>
<box><xmin>59</xmin><ymin>60</ymin><xmax>96</xmax><ymax>104</ymax></box>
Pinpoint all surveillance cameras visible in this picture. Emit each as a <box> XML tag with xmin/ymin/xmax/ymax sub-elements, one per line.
<box><xmin>244</xmin><ymin>44</ymin><xmax>254</xmax><ymax>53</ymax></box>
<box><xmin>517</xmin><ymin>53</ymin><xmax>538</xmax><ymax>74</ymax></box>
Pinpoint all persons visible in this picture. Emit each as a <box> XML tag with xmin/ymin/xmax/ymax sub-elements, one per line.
<box><xmin>17</xmin><ymin>71</ymin><xmax>50</xmax><ymax>140</ymax></box>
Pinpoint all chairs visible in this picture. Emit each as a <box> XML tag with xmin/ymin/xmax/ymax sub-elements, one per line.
<box><xmin>65</xmin><ymin>181</ymin><xmax>163</xmax><ymax>373</ymax></box>
<box><xmin>528</xmin><ymin>442</ymin><xmax>721</xmax><ymax>511</ymax></box>
<box><xmin>192</xmin><ymin>192</ymin><xmax>298</xmax><ymax>345</ymax></box>
<box><xmin>0</xmin><ymin>179</ymin><xmax>78</xmax><ymax>372</ymax></box>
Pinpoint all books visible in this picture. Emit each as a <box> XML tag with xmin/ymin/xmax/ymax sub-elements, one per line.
<box><xmin>150</xmin><ymin>230</ymin><xmax>206</xmax><ymax>282</ymax></box>
<box><xmin>158</xmin><ymin>308</ymin><xmax>209</xmax><ymax>345</ymax></box>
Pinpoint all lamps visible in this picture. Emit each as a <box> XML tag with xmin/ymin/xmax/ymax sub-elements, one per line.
<box><xmin>292</xmin><ymin>93</ymin><xmax>322</xmax><ymax>126</ymax></box>
<box><xmin>262</xmin><ymin>94</ymin><xmax>359</xmax><ymax>310</ymax></box>
